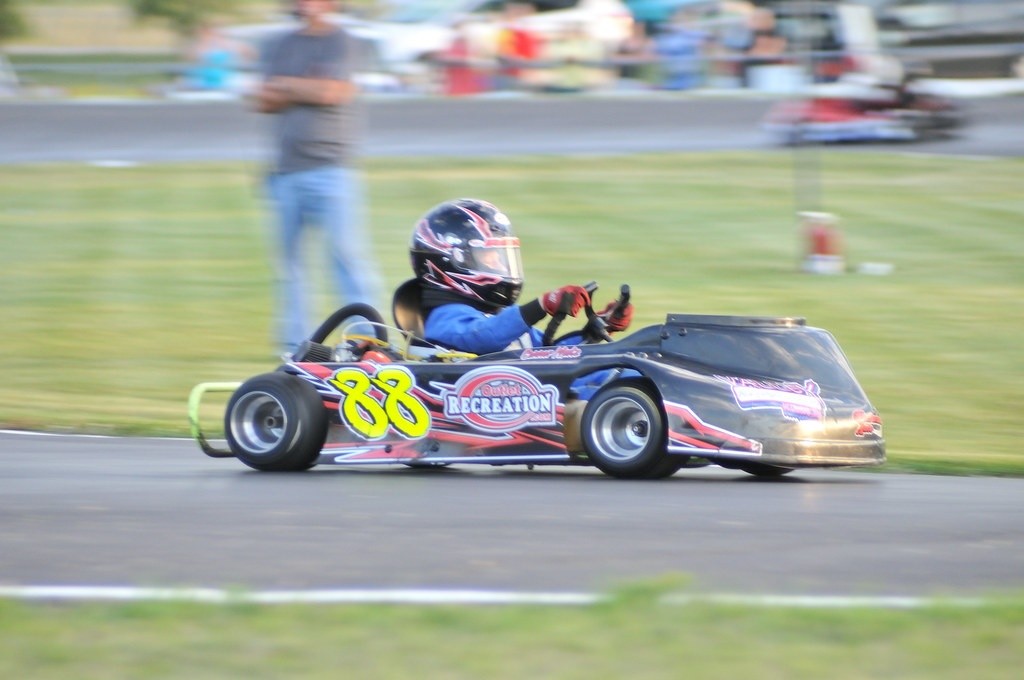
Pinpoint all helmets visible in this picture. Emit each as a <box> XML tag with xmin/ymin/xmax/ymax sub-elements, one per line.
<box><xmin>407</xmin><ymin>197</ymin><xmax>523</xmax><ymax>308</ymax></box>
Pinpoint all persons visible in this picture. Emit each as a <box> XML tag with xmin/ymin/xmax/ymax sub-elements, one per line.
<box><xmin>408</xmin><ymin>197</ymin><xmax>643</xmax><ymax>402</ymax></box>
<box><xmin>246</xmin><ymin>0</ymin><xmax>384</xmax><ymax>357</ymax></box>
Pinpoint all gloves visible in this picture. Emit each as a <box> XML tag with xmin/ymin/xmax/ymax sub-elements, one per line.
<box><xmin>596</xmin><ymin>301</ymin><xmax>633</xmax><ymax>331</ymax></box>
<box><xmin>544</xmin><ymin>285</ymin><xmax>591</xmax><ymax>317</ymax></box>
